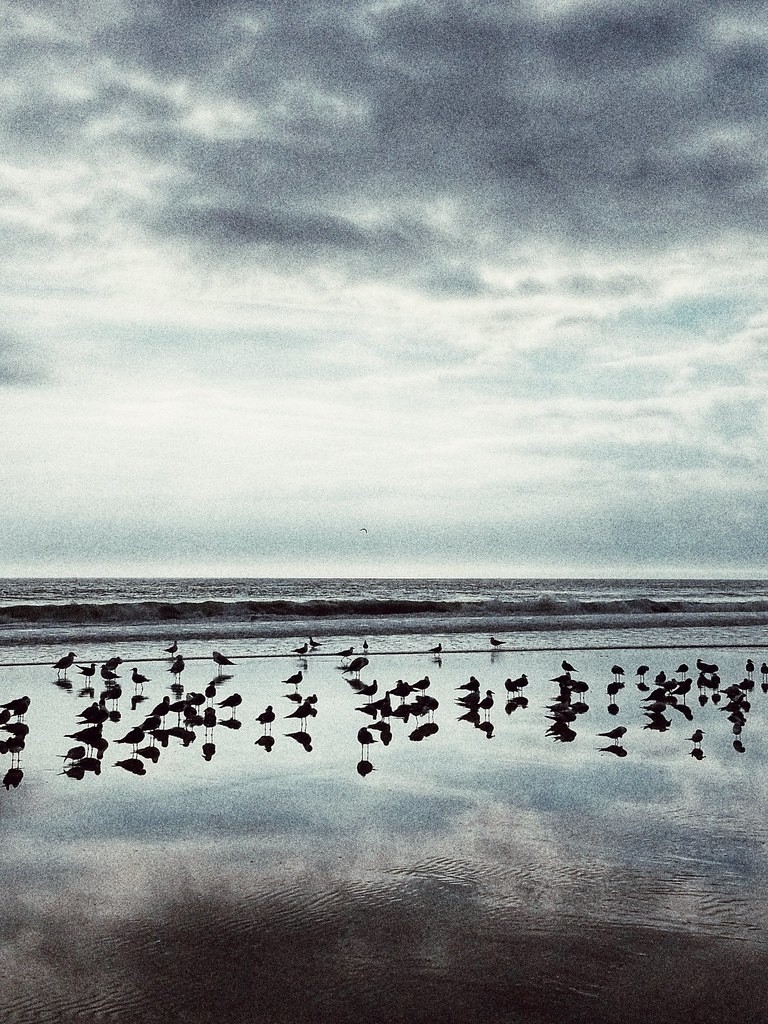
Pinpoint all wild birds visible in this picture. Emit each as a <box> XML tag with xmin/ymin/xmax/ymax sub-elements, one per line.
<box><xmin>361</xmin><ymin>528</ymin><xmax>368</xmax><ymax>533</ymax></box>
<box><xmin>0</xmin><ymin>636</ymin><xmax>767</xmax><ymax>791</ymax></box>
